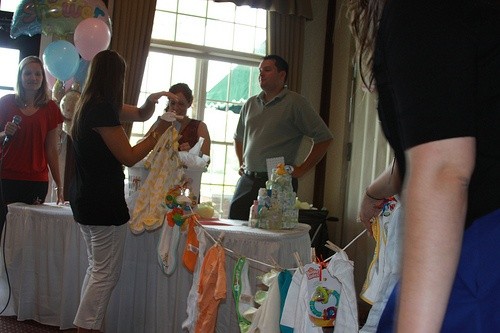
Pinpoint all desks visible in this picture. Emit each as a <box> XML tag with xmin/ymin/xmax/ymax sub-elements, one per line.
<box><xmin>0</xmin><ymin>203</ymin><xmax>311</xmax><ymax>333</ymax></box>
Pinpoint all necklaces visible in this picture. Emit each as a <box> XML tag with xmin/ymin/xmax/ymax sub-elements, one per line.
<box><xmin>24</xmin><ymin>103</ymin><xmax>33</xmax><ymax>108</ymax></box>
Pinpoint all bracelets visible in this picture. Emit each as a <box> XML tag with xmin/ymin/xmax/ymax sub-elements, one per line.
<box><xmin>366</xmin><ymin>190</ymin><xmax>385</xmax><ymax>200</ymax></box>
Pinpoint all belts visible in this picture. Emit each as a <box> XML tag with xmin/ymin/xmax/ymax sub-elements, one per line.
<box><xmin>243</xmin><ymin>169</ymin><xmax>268</xmax><ymax>182</ymax></box>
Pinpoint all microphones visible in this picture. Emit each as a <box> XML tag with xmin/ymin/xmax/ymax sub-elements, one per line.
<box><xmin>3</xmin><ymin>115</ymin><xmax>22</xmax><ymax>146</ymax></box>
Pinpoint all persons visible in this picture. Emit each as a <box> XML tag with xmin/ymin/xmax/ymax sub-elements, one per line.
<box><xmin>0</xmin><ymin>56</ymin><xmax>65</xmax><ymax>237</ymax></box>
<box><xmin>66</xmin><ymin>49</ymin><xmax>183</xmax><ymax>333</ymax></box>
<box><xmin>346</xmin><ymin>0</ymin><xmax>500</xmax><ymax>333</ymax></box>
<box><xmin>228</xmin><ymin>55</ymin><xmax>333</xmax><ymax>221</ymax></box>
<box><xmin>163</xmin><ymin>83</ymin><xmax>210</xmax><ymax>165</ymax></box>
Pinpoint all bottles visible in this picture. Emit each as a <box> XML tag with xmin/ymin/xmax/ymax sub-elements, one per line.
<box><xmin>257</xmin><ymin>187</ymin><xmax>270</xmax><ymax>228</ymax></box>
<box><xmin>248</xmin><ymin>200</ymin><xmax>259</xmax><ymax>228</ymax></box>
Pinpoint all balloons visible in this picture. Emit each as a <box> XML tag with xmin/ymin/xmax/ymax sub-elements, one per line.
<box><xmin>71</xmin><ymin>59</ymin><xmax>89</xmax><ymax>89</ymax></box>
<box><xmin>42</xmin><ymin>40</ymin><xmax>80</xmax><ymax>81</ymax></box>
<box><xmin>60</xmin><ymin>91</ymin><xmax>82</xmax><ymax>120</ymax></box>
<box><xmin>74</xmin><ymin>18</ymin><xmax>111</xmax><ymax>62</ymax></box>
